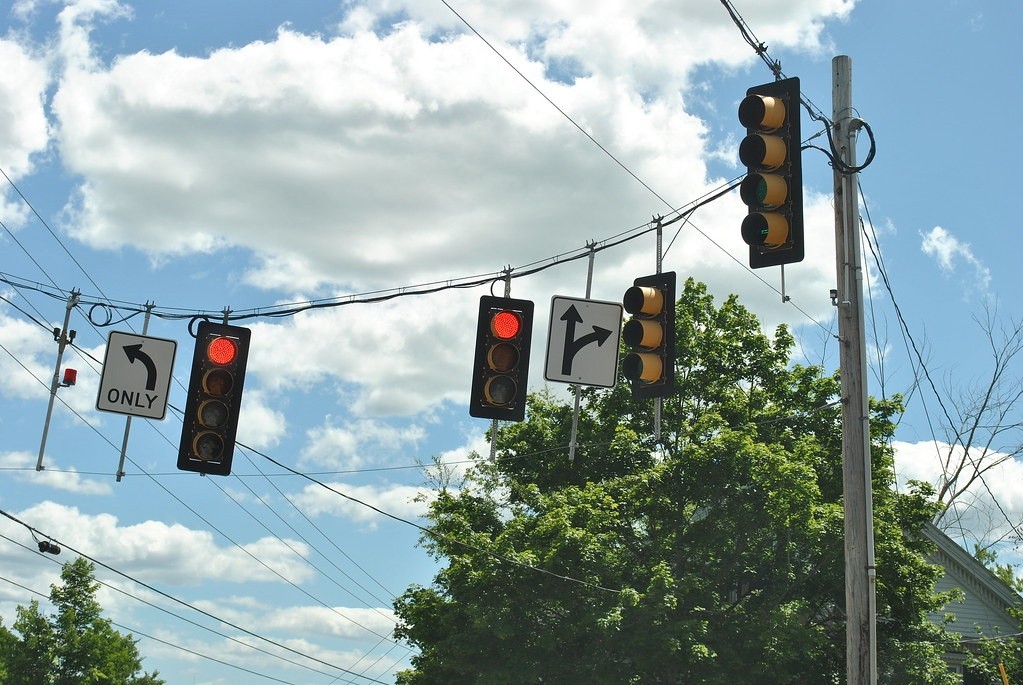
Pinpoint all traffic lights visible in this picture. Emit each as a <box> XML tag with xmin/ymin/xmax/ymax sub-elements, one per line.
<box><xmin>622</xmin><ymin>272</ymin><xmax>677</xmax><ymax>398</ymax></box>
<box><xmin>175</xmin><ymin>320</ymin><xmax>252</xmax><ymax>479</ymax></box>
<box><xmin>466</xmin><ymin>295</ymin><xmax>536</xmax><ymax>422</ymax></box>
<box><xmin>740</xmin><ymin>77</ymin><xmax>807</xmax><ymax>267</ymax></box>
